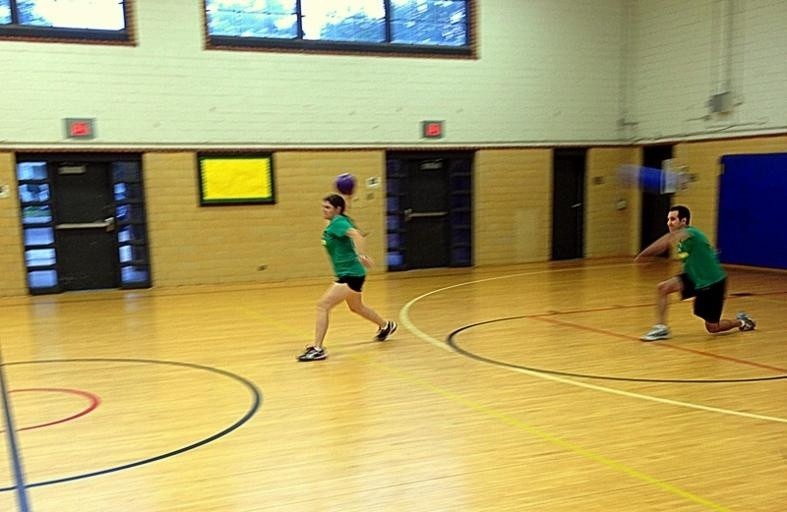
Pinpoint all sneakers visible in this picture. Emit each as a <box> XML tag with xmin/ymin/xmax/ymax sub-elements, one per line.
<box><xmin>736</xmin><ymin>312</ymin><xmax>756</xmax><ymax>331</ymax></box>
<box><xmin>299</xmin><ymin>343</ymin><xmax>327</xmax><ymax>361</ymax></box>
<box><xmin>376</xmin><ymin>321</ymin><xmax>397</xmax><ymax>340</ymax></box>
<box><xmin>641</xmin><ymin>323</ymin><xmax>669</xmax><ymax>341</ymax></box>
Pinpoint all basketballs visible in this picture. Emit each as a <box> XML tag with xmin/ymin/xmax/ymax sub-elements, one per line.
<box><xmin>336</xmin><ymin>173</ymin><xmax>356</xmax><ymax>194</ymax></box>
<box><xmin>638</xmin><ymin>167</ymin><xmax>667</xmax><ymax>192</ymax></box>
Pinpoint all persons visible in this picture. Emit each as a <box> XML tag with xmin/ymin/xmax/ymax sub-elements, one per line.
<box><xmin>296</xmin><ymin>195</ymin><xmax>397</xmax><ymax>361</ymax></box>
<box><xmin>634</xmin><ymin>206</ymin><xmax>756</xmax><ymax>341</ymax></box>
